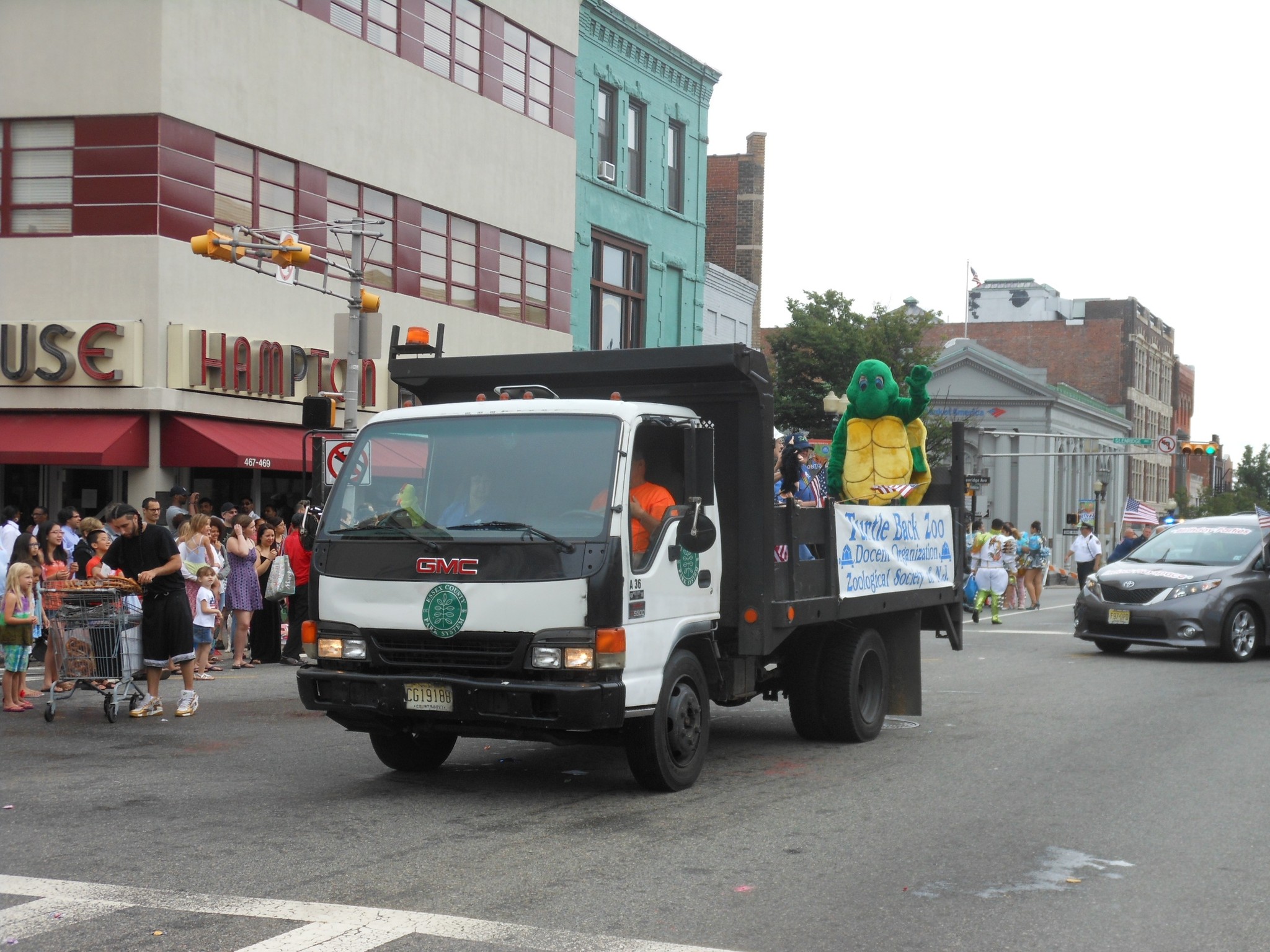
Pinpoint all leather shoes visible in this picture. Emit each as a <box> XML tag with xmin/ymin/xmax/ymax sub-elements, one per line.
<box><xmin>279</xmin><ymin>655</ymin><xmax>306</xmax><ymax>665</ymax></box>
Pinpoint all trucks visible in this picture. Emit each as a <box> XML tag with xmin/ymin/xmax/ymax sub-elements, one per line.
<box><xmin>294</xmin><ymin>345</ymin><xmax>963</xmax><ymax>793</ymax></box>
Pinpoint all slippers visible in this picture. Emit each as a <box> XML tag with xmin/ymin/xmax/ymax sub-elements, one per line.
<box><xmin>3</xmin><ymin>674</ymin><xmax>118</xmax><ymax>712</ymax></box>
<box><xmin>160</xmin><ymin>649</ymin><xmax>260</xmax><ymax>680</ymax></box>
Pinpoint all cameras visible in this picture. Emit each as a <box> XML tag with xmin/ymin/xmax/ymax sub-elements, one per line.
<box><xmin>195</xmin><ymin>494</ymin><xmax>199</xmax><ymax>499</ymax></box>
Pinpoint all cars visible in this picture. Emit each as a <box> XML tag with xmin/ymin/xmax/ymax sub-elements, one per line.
<box><xmin>1072</xmin><ymin>513</ymin><xmax>1270</xmax><ymax>662</ymax></box>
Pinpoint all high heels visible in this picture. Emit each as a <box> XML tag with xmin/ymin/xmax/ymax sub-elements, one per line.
<box><xmin>1027</xmin><ymin>601</ymin><xmax>1040</xmax><ymax>610</ymax></box>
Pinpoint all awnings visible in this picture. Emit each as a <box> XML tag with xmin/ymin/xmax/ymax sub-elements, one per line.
<box><xmin>159</xmin><ymin>411</ymin><xmax>429</xmax><ymax>478</ymax></box>
<box><xmin>0</xmin><ymin>409</ymin><xmax>150</xmax><ymax>468</ymax></box>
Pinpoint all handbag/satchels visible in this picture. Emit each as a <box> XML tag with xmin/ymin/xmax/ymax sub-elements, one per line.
<box><xmin>264</xmin><ymin>538</ymin><xmax>295</xmax><ymax>602</ymax></box>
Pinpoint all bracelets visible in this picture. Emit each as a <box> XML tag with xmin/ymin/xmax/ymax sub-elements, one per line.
<box><xmin>188</xmin><ymin>502</ymin><xmax>194</xmax><ymax>504</ymax></box>
<box><xmin>266</xmin><ymin>558</ymin><xmax>272</xmax><ymax>562</ymax></box>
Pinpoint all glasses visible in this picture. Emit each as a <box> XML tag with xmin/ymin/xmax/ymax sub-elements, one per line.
<box><xmin>178</xmin><ymin>493</ymin><xmax>187</xmax><ymax>496</ymax></box>
<box><xmin>31</xmin><ymin>513</ymin><xmax>43</xmax><ymax>518</ymax></box>
<box><xmin>29</xmin><ymin>542</ymin><xmax>40</xmax><ymax>548</ymax></box>
<box><xmin>224</xmin><ymin>511</ymin><xmax>238</xmax><ymax>515</ymax></box>
<box><xmin>240</xmin><ymin>502</ymin><xmax>252</xmax><ymax>506</ymax></box>
<box><xmin>48</xmin><ymin>530</ymin><xmax>64</xmax><ymax>536</ymax></box>
<box><xmin>146</xmin><ymin>508</ymin><xmax>162</xmax><ymax>513</ymax></box>
<box><xmin>775</xmin><ymin>441</ymin><xmax>783</xmax><ymax>445</ymax></box>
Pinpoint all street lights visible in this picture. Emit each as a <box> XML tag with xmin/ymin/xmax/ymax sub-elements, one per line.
<box><xmin>1092</xmin><ymin>478</ymin><xmax>1103</xmax><ymax>536</ymax></box>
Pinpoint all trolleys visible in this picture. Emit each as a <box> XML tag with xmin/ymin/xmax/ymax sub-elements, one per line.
<box><xmin>38</xmin><ymin>579</ymin><xmax>146</xmax><ymax>722</ymax></box>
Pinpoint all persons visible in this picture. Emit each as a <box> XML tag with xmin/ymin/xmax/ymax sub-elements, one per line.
<box><xmin>586</xmin><ymin>444</ymin><xmax>679</xmax><ymax>569</ymax></box>
<box><xmin>965</xmin><ymin>518</ymin><xmax>1047</xmax><ymax>625</ymax></box>
<box><xmin>1064</xmin><ymin>523</ymin><xmax>1102</xmax><ymax>591</ymax></box>
<box><xmin>1118</xmin><ymin>526</ymin><xmax>1167</xmax><ymax>560</ymax></box>
<box><xmin>773</xmin><ymin>426</ymin><xmax>827</xmax><ymax>560</ymax></box>
<box><xmin>0</xmin><ymin>486</ymin><xmax>379</xmax><ymax>717</ymax></box>
<box><xmin>435</xmin><ymin>467</ymin><xmax>508</xmax><ymax>530</ymax></box>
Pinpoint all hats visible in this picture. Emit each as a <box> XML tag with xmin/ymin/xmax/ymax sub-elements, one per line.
<box><xmin>170</xmin><ymin>486</ymin><xmax>191</xmax><ymax>497</ymax></box>
<box><xmin>1081</xmin><ymin>522</ymin><xmax>1092</xmax><ymax>529</ymax></box>
<box><xmin>773</xmin><ymin>426</ymin><xmax>784</xmax><ymax>441</ymax></box>
<box><xmin>220</xmin><ymin>502</ymin><xmax>238</xmax><ymax>512</ymax></box>
<box><xmin>784</xmin><ymin>432</ymin><xmax>814</xmax><ymax>453</ymax></box>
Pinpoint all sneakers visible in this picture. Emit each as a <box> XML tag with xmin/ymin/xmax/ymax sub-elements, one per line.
<box><xmin>130</xmin><ymin>693</ymin><xmax>163</xmax><ymax>716</ymax></box>
<box><xmin>176</xmin><ymin>690</ymin><xmax>199</xmax><ymax>716</ymax></box>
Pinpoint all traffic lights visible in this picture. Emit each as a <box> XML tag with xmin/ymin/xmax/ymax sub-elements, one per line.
<box><xmin>271</xmin><ymin>240</ymin><xmax>311</xmax><ymax>269</ymax></box>
<box><xmin>1067</xmin><ymin>513</ymin><xmax>1078</xmax><ymax>524</ymax></box>
<box><xmin>190</xmin><ymin>229</ymin><xmax>246</xmax><ymax>264</ymax></box>
<box><xmin>1182</xmin><ymin>442</ymin><xmax>1220</xmax><ymax>456</ymax></box>
<box><xmin>359</xmin><ymin>287</ymin><xmax>381</xmax><ymax>315</ymax></box>
<box><xmin>302</xmin><ymin>396</ymin><xmax>337</xmax><ymax>429</ymax></box>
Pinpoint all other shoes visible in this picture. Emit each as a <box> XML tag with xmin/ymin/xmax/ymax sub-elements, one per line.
<box><xmin>214</xmin><ymin>639</ymin><xmax>225</xmax><ymax>650</ymax></box>
<box><xmin>1010</xmin><ymin>603</ymin><xmax>1016</xmax><ymax>610</ymax></box>
<box><xmin>1001</xmin><ymin>604</ymin><xmax>1010</xmax><ymax>610</ymax></box>
<box><xmin>1017</xmin><ymin>603</ymin><xmax>1025</xmax><ymax>609</ymax></box>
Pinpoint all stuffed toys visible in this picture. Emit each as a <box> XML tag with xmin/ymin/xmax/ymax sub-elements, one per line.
<box><xmin>827</xmin><ymin>359</ymin><xmax>932</xmax><ymax>504</ymax></box>
<box><xmin>396</xmin><ymin>484</ymin><xmax>425</xmax><ymax>527</ymax></box>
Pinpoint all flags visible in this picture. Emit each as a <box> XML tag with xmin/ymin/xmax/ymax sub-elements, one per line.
<box><xmin>810</xmin><ymin>464</ymin><xmax>828</xmax><ymax>508</ymax></box>
<box><xmin>1123</xmin><ymin>497</ymin><xmax>1160</xmax><ymax>525</ymax></box>
<box><xmin>870</xmin><ymin>483</ymin><xmax>919</xmax><ymax>498</ymax></box>
<box><xmin>774</xmin><ymin>545</ymin><xmax>788</xmax><ymax>563</ymax></box>
<box><xmin>1255</xmin><ymin>505</ymin><xmax>1270</xmax><ymax>528</ymax></box>
<box><xmin>971</xmin><ymin>266</ymin><xmax>982</xmax><ymax>287</ymax></box>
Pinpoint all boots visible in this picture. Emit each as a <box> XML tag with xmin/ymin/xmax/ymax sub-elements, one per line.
<box><xmin>973</xmin><ymin>591</ymin><xmax>990</xmax><ymax>623</ymax></box>
<box><xmin>990</xmin><ymin>591</ymin><xmax>1003</xmax><ymax>623</ymax></box>
